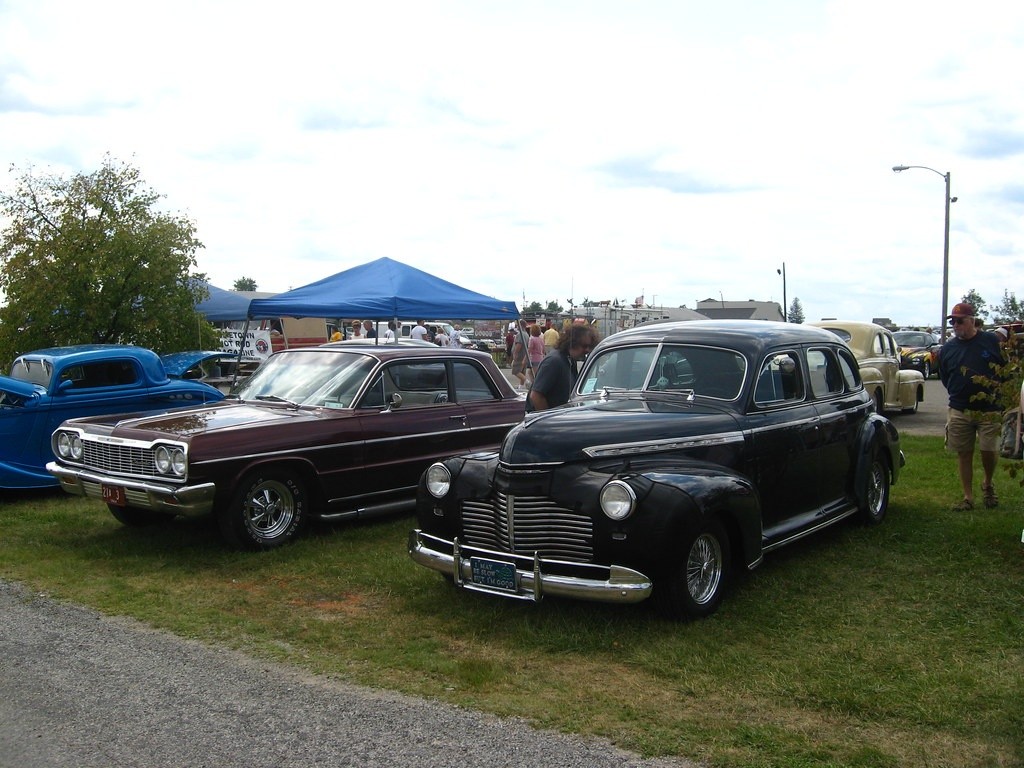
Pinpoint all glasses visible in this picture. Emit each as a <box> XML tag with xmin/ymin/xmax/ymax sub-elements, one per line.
<box><xmin>577</xmin><ymin>341</ymin><xmax>592</xmax><ymax>350</ymax></box>
<box><xmin>949</xmin><ymin>318</ymin><xmax>970</xmax><ymax>325</ymax></box>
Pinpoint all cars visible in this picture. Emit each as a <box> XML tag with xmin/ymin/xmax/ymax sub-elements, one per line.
<box><xmin>316</xmin><ymin>338</ymin><xmax>445</xmax><ymax>390</ymax></box>
<box><xmin>408</xmin><ymin>318</ymin><xmax>907</xmax><ymax>620</ymax></box>
<box><xmin>891</xmin><ymin>330</ymin><xmax>939</xmax><ymax>380</ymax></box>
<box><xmin>0</xmin><ymin>342</ymin><xmax>240</xmax><ymax>490</ymax></box>
<box><xmin>770</xmin><ymin>317</ymin><xmax>925</xmax><ymax>418</ymax></box>
<box><xmin>469</xmin><ymin>338</ymin><xmax>507</xmax><ymax>350</ymax></box>
<box><xmin>44</xmin><ymin>346</ymin><xmax>529</xmax><ymax>552</ymax></box>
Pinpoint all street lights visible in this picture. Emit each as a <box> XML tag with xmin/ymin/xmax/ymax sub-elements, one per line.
<box><xmin>777</xmin><ymin>262</ymin><xmax>786</xmax><ymax>322</ymax></box>
<box><xmin>892</xmin><ymin>163</ymin><xmax>959</xmax><ymax>347</ymax></box>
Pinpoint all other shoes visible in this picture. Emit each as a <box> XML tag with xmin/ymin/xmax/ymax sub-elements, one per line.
<box><xmin>526</xmin><ymin>381</ymin><xmax>532</xmax><ymax>390</ymax></box>
<box><xmin>981</xmin><ymin>484</ymin><xmax>998</xmax><ymax>508</ymax></box>
<box><xmin>953</xmin><ymin>499</ymin><xmax>974</xmax><ymax>513</ymax></box>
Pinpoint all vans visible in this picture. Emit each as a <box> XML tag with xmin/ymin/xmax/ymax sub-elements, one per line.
<box><xmin>359</xmin><ymin>322</ymin><xmax>473</xmax><ymax>350</ymax></box>
<box><xmin>459</xmin><ymin>328</ymin><xmax>475</xmax><ymax>336</ymax></box>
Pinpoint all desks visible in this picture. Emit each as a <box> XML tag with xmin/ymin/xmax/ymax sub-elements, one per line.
<box><xmin>468</xmin><ymin>346</ymin><xmax>506</xmax><ymax>366</ymax></box>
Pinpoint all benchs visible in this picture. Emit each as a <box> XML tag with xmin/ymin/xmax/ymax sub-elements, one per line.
<box><xmin>701</xmin><ymin>370</ymin><xmax>805</xmax><ymax>397</ymax></box>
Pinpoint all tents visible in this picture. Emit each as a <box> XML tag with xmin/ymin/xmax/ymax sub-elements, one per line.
<box><xmin>177</xmin><ymin>276</ymin><xmax>288</xmax><ymax>348</ymax></box>
<box><xmin>229</xmin><ymin>257</ymin><xmax>535</xmax><ymax>394</ymax></box>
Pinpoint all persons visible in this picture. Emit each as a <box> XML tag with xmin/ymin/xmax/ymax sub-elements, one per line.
<box><xmin>271</xmin><ymin>323</ymin><xmax>280</xmax><ymax>336</ymax></box>
<box><xmin>450</xmin><ymin>324</ymin><xmax>460</xmax><ymax>345</ymax></box>
<box><xmin>363</xmin><ymin>320</ymin><xmax>376</xmax><ymax>338</ymax></box>
<box><xmin>946</xmin><ymin>331</ymin><xmax>954</xmax><ymax>341</ymax></box>
<box><xmin>925</xmin><ymin>327</ymin><xmax>937</xmax><ymax>345</ymax></box>
<box><xmin>424</xmin><ymin>324</ymin><xmax>449</xmax><ymax>346</ymax></box>
<box><xmin>329</xmin><ymin>326</ymin><xmax>342</xmax><ymax>342</ymax></box>
<box><xmin>938</xmin><ymin>303</ymin><xmax>1010</xmax><ymax>511</ymax></box>
<box><xmin>523</xmin><ymin>324</ymin><xmax>599</xmax><ymax>414</ymax></box>
<box><xmin>384</xmin><ymin>321</ymin><xmax>393</xmax><ymax>338</ymax></box>
<box><xmin>974</xmin><ymin>319</ymin><xmax>1008</xmax><ymax>342</ymax></box>
<box><xmin>410</xmin><ymin>320</ymin><xmax>427</xmax><ymax>340</ymax></box>
<box><xmin>506</xmin><ymin>319</ymin><xmax>559</xmax><ymax>389</ymax></box>
<box><xmin>348</xmin><ymin>320</ymin><xmax>365</xmax><ymax>339</ymax></box>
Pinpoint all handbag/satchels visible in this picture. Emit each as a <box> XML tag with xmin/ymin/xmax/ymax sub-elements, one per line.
<box><xmin>1000</xmin><ymin>399</ymin><xmax>1024</xmax><ymax>460</ymax></box>
<box><xmin>525</xmin><ymin>392</ymin><xmax>565</xmax><ymax>413</ymax></box>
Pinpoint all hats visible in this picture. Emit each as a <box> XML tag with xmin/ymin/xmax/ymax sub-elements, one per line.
<box><xmin>995</xmin><ymin>328</ymin><xmax>1007</xmax><ymax>338</ymax></box>
<box><xmin>516</xmin><ymin>320</ymin><xmax>527</xmax><ymax>326</ymax></box>
<box><xmin>947</xmin><ymin>303</ymin><xmax>974</xmax><ymax>319</ymax></box>
<box><xmin>352</xmin><ymin>320</ymin><xmax>361</xmax><ymax>326</ymax></box>
